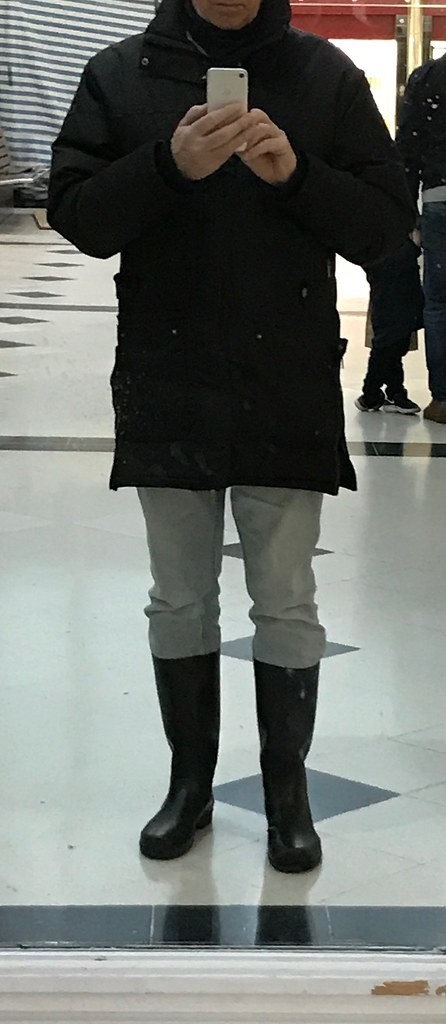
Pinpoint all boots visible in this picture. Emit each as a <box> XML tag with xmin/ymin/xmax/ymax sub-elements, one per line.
<box><xmin>250</xmin><ymin>658</ymin><xmax>322</xmax><ymax>874</ymax></box>
<box><xmin>140</xmin><ymin>647</ymin><xmax>221</xmax><ymax>860</ymax></box>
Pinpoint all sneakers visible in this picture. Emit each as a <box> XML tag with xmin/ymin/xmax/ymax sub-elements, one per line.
<box><xmin>383</xmin><ymin>388</ymin><xmax>421</xmax><ymax>414</ymax></box>
<box><xmin>354</xmin><ymin>389</ymin><xmax>385</xmax><ymax>411</ymax></box>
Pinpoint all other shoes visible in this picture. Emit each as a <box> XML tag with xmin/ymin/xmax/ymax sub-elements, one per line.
<box><xmin>424</xmin><ymin>399</ymin><xmax>446</xmax><ymax>424</ymax></box>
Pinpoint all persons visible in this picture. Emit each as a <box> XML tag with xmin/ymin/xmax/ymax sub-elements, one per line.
<box><xmin>395</xmin><ymin>48</ymin><xmax>446</xmax><ymax>427</ymax></box>
<box><xmin>44</xmin><ymin>0</ymin><xmax>416</xmax><ymax>873</ymax></box>
<box><xmin>350</xmin><ymin>205</ymin><xmax>423</xmax><ymax>417</ymax></box>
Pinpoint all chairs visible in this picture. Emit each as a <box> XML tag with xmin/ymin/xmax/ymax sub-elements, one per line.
<box><xmin>0</xmin><ymin>127</ymin><xmax>60</xmax><ymax>230</ymax></box>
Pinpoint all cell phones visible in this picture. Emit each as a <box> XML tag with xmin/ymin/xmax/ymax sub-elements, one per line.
<box><xmin>207</xmin><ymin>68</ymin><xmax>248</xmax><ymax>151</ymax></box>
